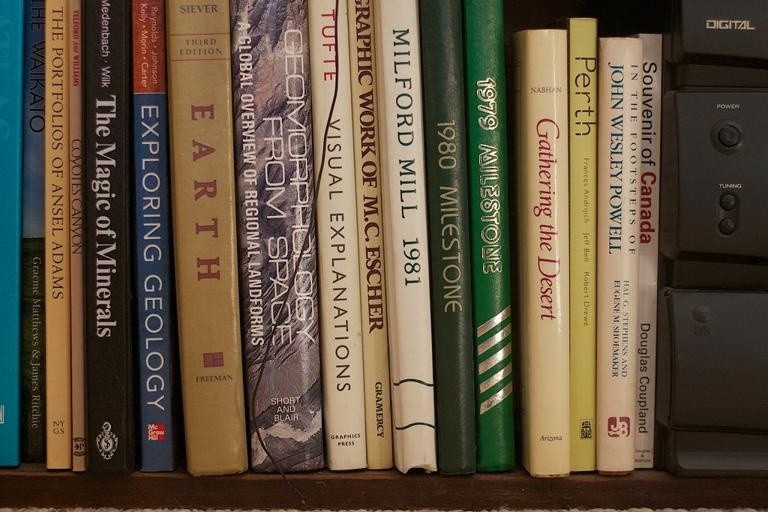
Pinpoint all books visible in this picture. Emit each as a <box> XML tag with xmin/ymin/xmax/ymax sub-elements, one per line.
<box><xmin>0</xmin><ymin>1</ymin><xmax>662</xmax><ymax>480</ymax></box>
